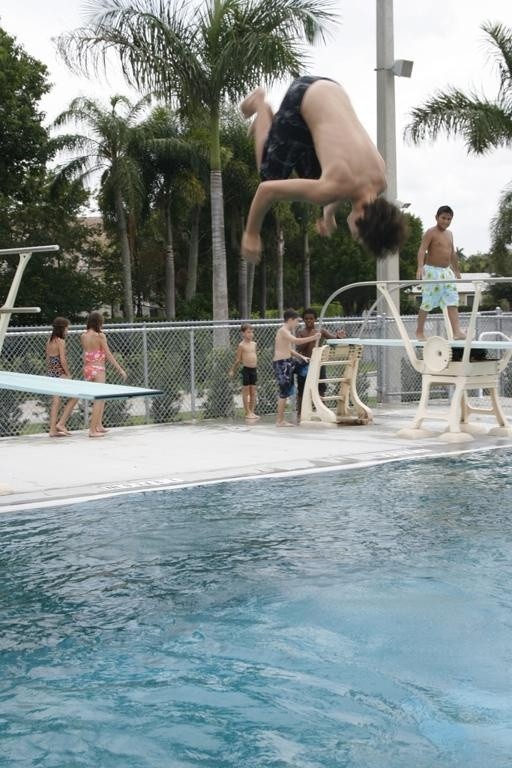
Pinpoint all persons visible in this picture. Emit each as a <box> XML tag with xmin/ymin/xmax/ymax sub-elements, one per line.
<box><xmin>45</xmin><ymin>317</ymin><xmax>79</xmax><ymax>437</ymax></box>
<box><xmin>80</xmin><ymin>313</ymin><xmax>128</xmax><ymax>437</ymax></box>
<box><xmin>414</xmin><ymin>206</ymin><xmax>466</xmax><ymax>342</ymax></box>
<box><xmin>295</xmin><ymin>308</ymin><xmax>347</xmax><ymax>426</ymax></box>
<box><xmin>228</xmin><ymin>323</ymin><xmax>260</xmax><ymax>419</ymax></box>
<box><xmin>272</xmin><ymin>308</ymin><xmax>321</xmax><ymax>426</ymax></box>
<box><xmin>238</xmin><ymin>75</ymin><xmax>414</xmax><ymax>268</ymax></box>
